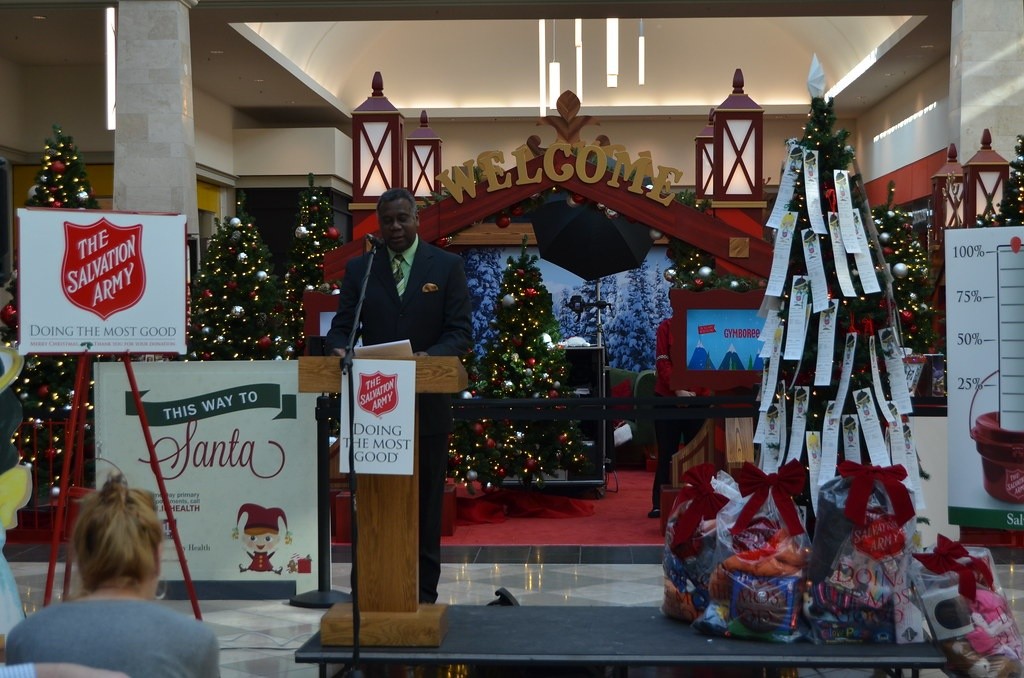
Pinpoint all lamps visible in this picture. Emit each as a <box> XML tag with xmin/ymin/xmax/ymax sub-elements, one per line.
<box><xmin>350</xmin><ymin>76</ymin><xmax>407</xmax><ymax>200</ymax></box>
<box><xmin>709</xmin><ymin>70</ymin><xmax>772</xmax><ymax>203</ymax></box>
<box><xmin>407</xmin><ymin>108</ymin><xmax>442</xmax><ymax>199</ymax></box>
<box><xmin>105</xmin><ymin>6</ymin><xmax>116</xmax><ymax>131</ymax></box>
<box><xmin>695</xmin><ymin>108</ymin><xmax>714</xmax><ymax>199</ymax></box>
<box><xmin>540</xmin><ymin>19</ymin><xmax>646</xmax><ymax>118</ymax></box>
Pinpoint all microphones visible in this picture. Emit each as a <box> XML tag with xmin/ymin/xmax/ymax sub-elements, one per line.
<box><xmin>366</xmin><ymin>234</ymin><xmax>384</xmax><ymax>250</ymax></box>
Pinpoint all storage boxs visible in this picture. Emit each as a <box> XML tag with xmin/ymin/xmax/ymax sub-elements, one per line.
<box><xmin>923</xmin><ymin>581</ymin><xmax>973</xmax><ymax>639</ymax></box>
<box><xmin>732</xmin><ymin>576</ymin><xmax>801</xmax><ymax>642</ymax></box>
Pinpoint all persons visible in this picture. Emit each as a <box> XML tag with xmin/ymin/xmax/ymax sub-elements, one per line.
<box><xmin>0</xmin><ymin>471</ymin><xmax>222</xmax><ymax>677</ymax></box>
<box><xmin>324</xmin><ymin>187</ymin><xmax>475</xmax><ymax>605</ymax></box>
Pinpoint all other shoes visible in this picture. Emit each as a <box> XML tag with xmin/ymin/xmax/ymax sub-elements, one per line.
<box><xmin>648</xmin><ymin>505</ymin><xmax>660</xmax><ymax>518</ymax></box>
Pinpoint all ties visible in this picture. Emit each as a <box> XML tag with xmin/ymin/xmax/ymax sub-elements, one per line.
<box><xmin>393</xmin><ymin>255</ymin><xmax>405</xmax><ymax>304</ymax></box>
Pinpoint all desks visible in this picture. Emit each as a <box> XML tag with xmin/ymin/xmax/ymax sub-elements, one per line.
<box><xmin>295</xmin><ymin>603</ymin><xmax>947</xmax><ymax>678</ymax></box>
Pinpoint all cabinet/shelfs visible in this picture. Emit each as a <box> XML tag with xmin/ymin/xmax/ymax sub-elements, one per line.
<box><xmin>491</xmin><ymin>346</ymin><xmax>605</xmax><ymax>498</ymax></box>
